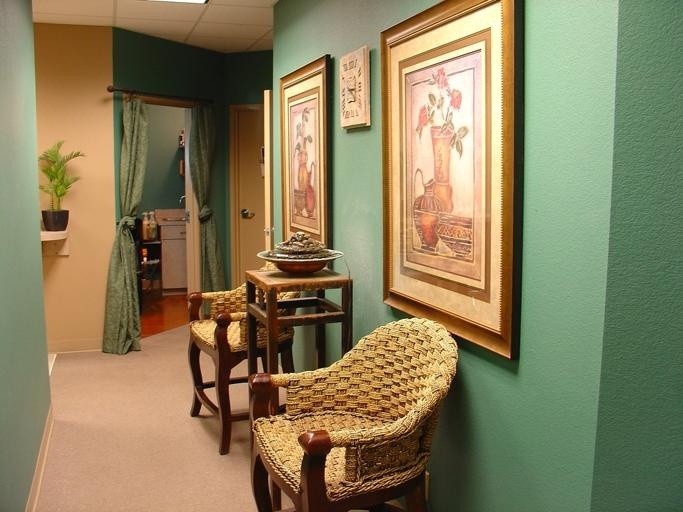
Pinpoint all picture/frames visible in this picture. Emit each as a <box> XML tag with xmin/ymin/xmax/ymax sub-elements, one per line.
<box><xmin>280</xmin><ymin>54</ymin><xmax>332</xmax><ymax>252</ymax></box>
<box><xmin>380</xmin><ymin>1</ymin><xmax>523</xmax><ymax>360</ymax></box>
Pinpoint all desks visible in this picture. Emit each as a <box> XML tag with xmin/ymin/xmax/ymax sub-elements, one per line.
<box><xmin>242</xmin><ymin>268</ymin><xmax>353</xmax><ymax>512</ymax></box>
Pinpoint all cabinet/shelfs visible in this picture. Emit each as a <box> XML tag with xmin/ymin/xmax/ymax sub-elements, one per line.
<box><xmin>159</xmin><ymin>226</ymin><xmax>188</xmax><ymax>290</ymax></box>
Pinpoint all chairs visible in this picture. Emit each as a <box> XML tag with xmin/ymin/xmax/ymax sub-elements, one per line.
<box><xmin>247</xmin><ymin>318</ymin><xmax>461</xmax><ymax>510</ymax></box>
<box><xmin>185</xmin><ymin>262</ymin><xmax>297</xmax><ymax>454</ymax></box>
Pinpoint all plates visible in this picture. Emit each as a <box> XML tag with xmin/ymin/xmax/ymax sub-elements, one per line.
<box><xmin>256</xmin><ymin>249</ymin><xmax>344</xmax><ymax>274</ymax></box>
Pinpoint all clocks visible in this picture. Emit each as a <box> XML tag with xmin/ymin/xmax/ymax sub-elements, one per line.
<box><xmin>339</xmin><ymin>44</ymin><xmax>369</xmax><ymax>130</ymax></box>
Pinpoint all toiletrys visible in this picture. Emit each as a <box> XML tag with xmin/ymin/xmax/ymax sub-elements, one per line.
<box><xmin>148</xmin><ymin>211</ymin><xmax>158</xmax><ymax>241</ymax></box>
<box><xmin>141</xmin><ymin>211</ymin><xmax>150</xmax><ymax>241</ymax></box>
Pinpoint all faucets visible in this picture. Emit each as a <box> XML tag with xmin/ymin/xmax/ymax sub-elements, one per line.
<box><xmin>178</xmin><ymin>195</ymin><xmax>186</xmax><ymax>206</ymax></box>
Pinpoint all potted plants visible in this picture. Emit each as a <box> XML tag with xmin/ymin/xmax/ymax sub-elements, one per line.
<box><xmin>36</xmin><ymin>137</ymin><xmax>85</xmax><ymax>230</ymax></box>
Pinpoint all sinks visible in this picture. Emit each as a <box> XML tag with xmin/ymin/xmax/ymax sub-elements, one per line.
<box><xmin>162</xmin><ymin>216</ymin><xmax>187</xmax><ymax>221</ymax></box>
